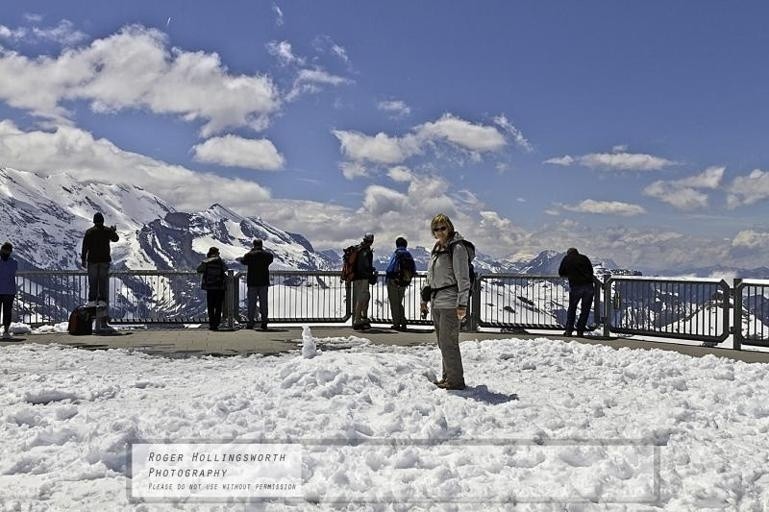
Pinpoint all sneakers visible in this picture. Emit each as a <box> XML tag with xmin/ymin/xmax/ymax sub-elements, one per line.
<box><xmin>434</xmin><ymin>380</ymin><xmax>445</xmax><ymax>384</ymax></box>
<box><xmin>97</xmin><ymin>299</ymin><xmax>108</xmax><ymax>307</ymax></box>
<box><xmin>83</xmin><ymin>300</ymin><xmax>97</xmax><ymax>308</ymax></box>
<box><xmin>439</xmin><ymin>383</ymin><xmax>465</xmax><ymax>390</ymax></box>
<box><xmin>3</xmin><ymin>332</ymin><xmax>12</xmax><ymax>339</ymax></box>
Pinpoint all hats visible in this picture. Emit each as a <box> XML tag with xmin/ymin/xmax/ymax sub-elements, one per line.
<box><xmin>363</xmin><ymin>233</ymin><xmax>374</xmax><ymax>243</ymax></box>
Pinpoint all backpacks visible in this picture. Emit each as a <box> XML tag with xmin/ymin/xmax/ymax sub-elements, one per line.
<box><xmin>393</xmin><ymin>251</ymin><xmax>413</xmax><ymax>286</ymax></box>
<box><xmin>430</xmin><ymin>239</ymin><xmax>477</xmax><ymax>294</ymax></box>
<box><xmin>205</xmin><ymin>255</ymin><xmax>224</xmax><ymax>286</ymax></box>
<box><xmin>340</xmin><ymin>244</ymin><xmax>366</xmax><ymax>283</ymax></box>
<box><xmin>68</xmin><ymin>304</ymin><xmax>91</xmax><ymax>335</ymax></box>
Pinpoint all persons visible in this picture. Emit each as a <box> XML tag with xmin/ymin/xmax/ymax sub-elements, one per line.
<box><xmin>197</xmin><ymin>246</ymin><xmax>233</xmax><ymax>331</ymax></box>
<box><xmin>419</xmin><ymin>212</ymin><xmax>474</xmax><ymax>391</ymax></box>
<box><xmin>239</xmin><ymin>238</ymin><xmax>274</xmax><ymax>331</ymax></box>
<box><xmin>1</xmin><ymin>241</ymin><xmax>18</xmax><ymax>341</ymax></box>
<box><xmin>382</xmin><ymin>235</ymin><xmax>418</xmax><ymax>331</ymax></box>
<box><xmin>341</xmin><ymin>231</ymin><xmax>376</xmax><ymax>332</ymax></box>
<box><xmin>557</xmin><ymin>247</ymin><xmax>595</xmax><ymax>336</ymax></box>
<box><xmin>80</xmin><ymin>212</ymin><xmax>120</xmax><ymax>327</ymax></box>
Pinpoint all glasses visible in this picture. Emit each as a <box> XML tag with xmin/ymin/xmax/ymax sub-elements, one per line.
<box><xmin>434</xmin><ymin>226</ymin><xmax>446</xmax><ymax>232</ymax></box>
